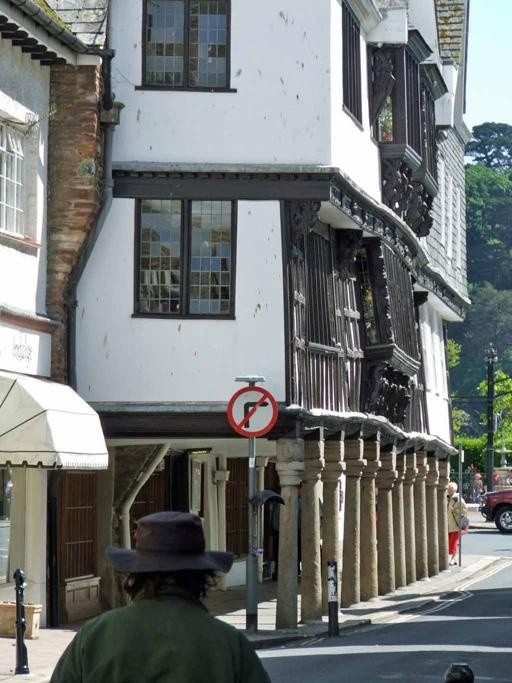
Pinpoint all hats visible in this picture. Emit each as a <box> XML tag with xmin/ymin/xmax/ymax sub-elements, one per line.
<box><xmin>103</xmin><ymin>508</ymin><xmax>238</xmax><ymax>576</ymax></box>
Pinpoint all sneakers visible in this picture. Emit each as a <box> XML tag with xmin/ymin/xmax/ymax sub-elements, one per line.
<box><xmin>448</xmin><ymin>560</ymin><xmax>459</xmax><ymax>566</ymax></box>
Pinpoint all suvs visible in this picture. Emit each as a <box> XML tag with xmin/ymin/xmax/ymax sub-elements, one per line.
<box><xmin>479</xmin><ymin>486</ymin><xmax>511</xmax><ymax>532</ymax></box>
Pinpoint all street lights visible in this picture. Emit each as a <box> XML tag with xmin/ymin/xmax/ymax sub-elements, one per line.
<box><xmin>479</xmin><ymin>343</ymin><xmax>497</xmax><ymax>492</ymax></box>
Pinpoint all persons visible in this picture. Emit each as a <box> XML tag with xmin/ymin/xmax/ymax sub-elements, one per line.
<box><xmin>473</xmin><ymin>474</ymin><xmax>483</xmax><ymax>504</ymax></box>
<box><xmin>445</xmin><ymin>482</ymin><xmax>469</xmax><ymax>565</ymax></box>
<box><xmin>51</xmin><ymin>511</ymin><xmax>272</xmax><ymax>683</ymax></box>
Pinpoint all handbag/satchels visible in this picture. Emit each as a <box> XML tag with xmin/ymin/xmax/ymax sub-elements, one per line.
<box><xmin>458</xmin><ymin>515</ymin><xmax>470</xmax><ymax>530</ymax></box>
